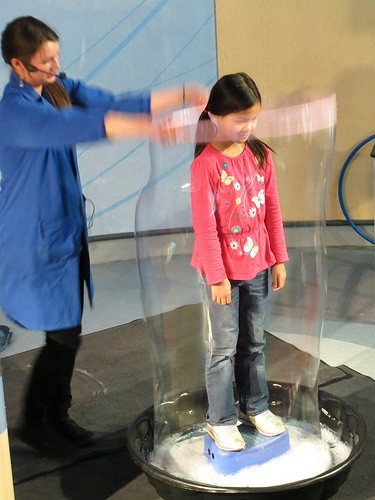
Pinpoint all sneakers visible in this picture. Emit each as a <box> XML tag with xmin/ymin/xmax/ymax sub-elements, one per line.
<box><xmin>206</xmin><ymin>422</ymin><xmax>245</xmax><ymax>452</ymax></box>
<box><xmin>237</xmin><ymin>407</ymin><xmax>286</xmax><ymax>436</ymax></box>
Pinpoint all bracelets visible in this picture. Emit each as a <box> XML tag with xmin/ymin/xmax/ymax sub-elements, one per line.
<box><xmin>182</xmin><ymin>84</ymin><xmax>185</xmax><ymax>106</ymax></box>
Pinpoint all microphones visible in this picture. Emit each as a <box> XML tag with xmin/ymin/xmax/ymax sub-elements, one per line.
<box><xmin>26</xmin><ymin>65</ymin><xmax>66</xmax><ymax>79</ymax></box>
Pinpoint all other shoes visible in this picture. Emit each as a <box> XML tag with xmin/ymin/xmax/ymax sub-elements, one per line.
<box><xmin>53</xmin><ymin>415</ymin><xmax>91</xmax><ymax>447</ymax></box>
<box><xmin>16</xmin><ymin>423</ymin><xmax>79</xmax><ymax>459</ymax></box>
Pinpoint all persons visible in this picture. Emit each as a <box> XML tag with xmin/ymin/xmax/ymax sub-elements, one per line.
<box><xmin>190</xmin><ymin>71</ymin><xmax>291</xmax><ymax>454</ymax></box>
<box><xmin>0</xmin><ymin>14</ymin><xmax>209</xmax><ymax>460</ymax></box>
